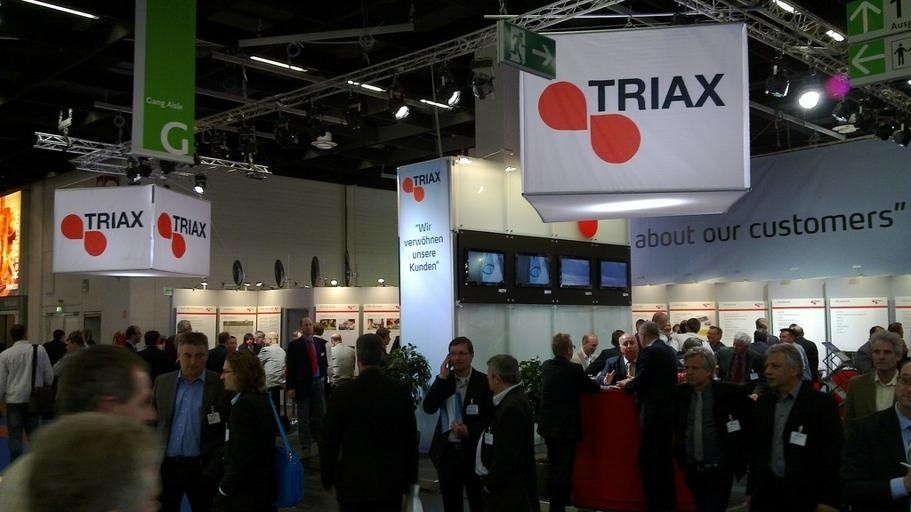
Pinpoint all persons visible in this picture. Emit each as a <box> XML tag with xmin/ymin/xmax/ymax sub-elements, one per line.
<box><xmin>1</xmin><ymin>317</ymin><xmax>418</xmax><ymax>512</ymax></box>
<box><xmin>422</xmin><ymin>311</ymin><xmax>911</xmax><ymax>512</ymax></box>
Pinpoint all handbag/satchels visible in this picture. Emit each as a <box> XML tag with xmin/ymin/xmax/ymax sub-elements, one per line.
<box><xmin>277</xmin><ymin>446</ymin><xmax>305</xmax><ymax>507</ymax></box>
<box><xmin>31</xmin><ymin>386</ymin><xmax>54</xmax><ymax>408</ymax></box>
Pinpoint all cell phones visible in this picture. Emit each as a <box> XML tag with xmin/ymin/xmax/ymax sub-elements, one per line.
<box><xmin>448</xmin><ymin>354</ymin><xmax>453</xmax><ymax>367</ymax></box>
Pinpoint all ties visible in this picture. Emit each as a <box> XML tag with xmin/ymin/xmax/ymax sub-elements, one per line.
<box><xmin>626</xmin><ymin>363</ymin><xmax>632</xmax><ymax>375</ymax></box>
<box><xmin>693</xmin><ymin>391</ymin><xmax>704</xmax><ymax>461</ymax></box>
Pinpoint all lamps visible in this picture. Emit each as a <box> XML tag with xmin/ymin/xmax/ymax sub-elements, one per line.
<box><xmin>388</xmin><ymin>95</ymin><xmax>410</xmax><ymax>120</ymax></box>
<box><xmin>468</xmin><ymin>68</ymin><xmax>496</xmax><ymax>99</ymax></box>
<box><xmin>765</xmin><ymin>54</ymin><xmax>826</xmax><ymax>115</ymax></box>
<box><xmin>193</xmin><ymin>175</ymin><xmax>206</xmax><ymax>194</ymax></box>
<box><xmin>311</xmin><ymin>130</ymin><xmax>338</xmax><ymax>151</ymax></box>
<box><xmin>431</xmin><ymin>82</ymin><xmax>461</xmax><ymax>107</ymax></box>
